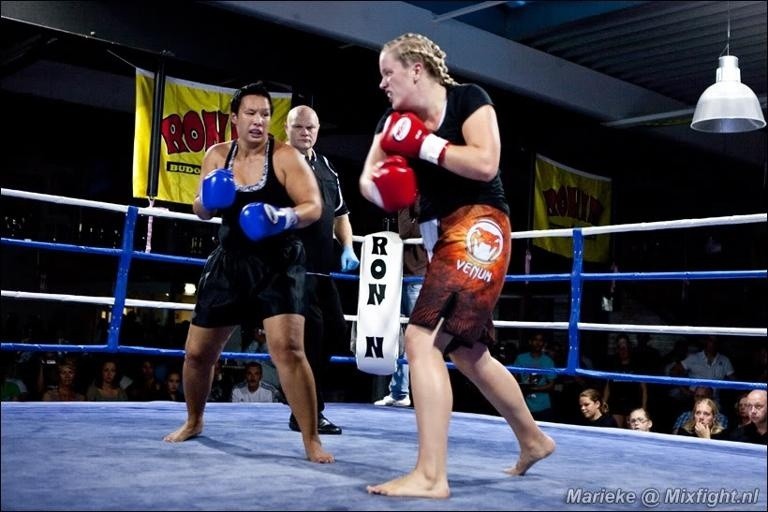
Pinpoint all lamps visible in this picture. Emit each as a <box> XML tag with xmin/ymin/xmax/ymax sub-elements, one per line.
<box><xmin>690</xmin><ymin>1</ymin><xmax>767</xmax><ymax>134</ymax></box>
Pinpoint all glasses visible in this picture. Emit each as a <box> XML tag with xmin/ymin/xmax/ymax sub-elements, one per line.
<box><xmin>630</xmin><ymin>418</ymin><xmax>648</xmax><ymax>426</ymax></box>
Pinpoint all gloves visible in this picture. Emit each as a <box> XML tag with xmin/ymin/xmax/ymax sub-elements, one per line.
<box><xmin>341</xmin><ymin>245</ymin><xmax>360</xmax><ymax>272</ymax></box>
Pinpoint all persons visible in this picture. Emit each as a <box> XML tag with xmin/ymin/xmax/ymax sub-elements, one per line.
<box><xmin>372</xmin><ymin>197</ymin><xmax>440</xmax><ymax>406</ymax></box>
<box><xmin>0</xmin><ymin>316</ymin><xmax>282</xmax><ymax>403</ymax></box>
<box><xmin>359</xmin><ymin>32</ymin><xmax>557</xmax><ymax>501</ymax></box>
<box><xmin>159</xmin><ymin>81</ymin><xmax>334</xmax><ymax>464</ymax></box>
<box><xmin>282</xmin><ymin>104</ymin><xmax>360</xmax><ymax>434</ymax></box>
<box><xmin>511</xmin><ymin>330</ymin><xmax>767</xmax><ymax>446</ymax></box>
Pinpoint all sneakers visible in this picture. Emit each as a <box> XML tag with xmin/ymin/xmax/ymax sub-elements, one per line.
<box><xmin>288</xmin><ymin>411</ymin><xmax>342</xmax><ymax>435</ymax></box>
<box><xmin>374</xmin><ymin>392</ymin><xmax>411</xmax><ymax>407</ymax></box>
